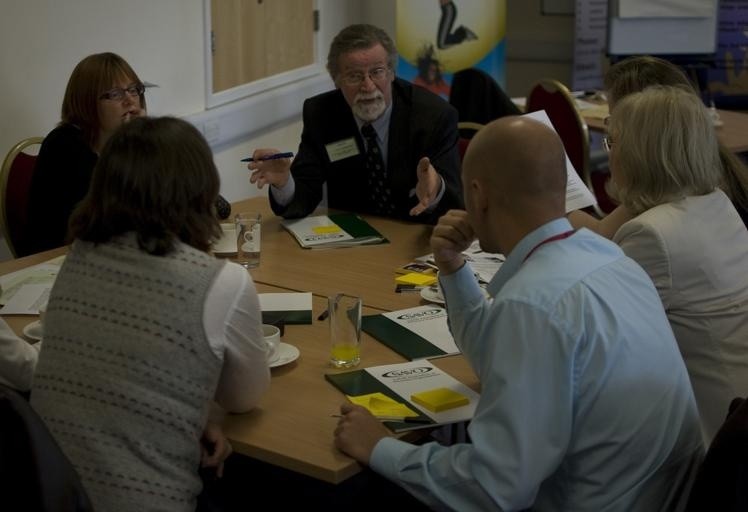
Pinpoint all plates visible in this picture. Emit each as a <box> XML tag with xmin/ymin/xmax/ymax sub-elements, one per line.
<box><xmin>268</xmin><ymin>343</ymin><xmax>300</xmax><ymax>368</ymax></box>
<box><xmin>421</xmin><ymin>284</ymin><xmax>490</xmax><ymax>304</ymax></box>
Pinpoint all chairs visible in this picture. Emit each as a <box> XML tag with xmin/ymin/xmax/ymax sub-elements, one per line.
<box><xmin>451</xmin><ymin>68</ymin><xmax>523</xmax><ymax>117</ymax></box>
<box><xmin>1</xmin><ymin>389</ymin><xmax>77</xmax><ymax>510</ymax></box>
<box><xmin>692</xmin><ymin>398</ymin><xmax>745</xmax><ymax>511</ymax></box>
<box><xmin>524</xmin><ymin>79</ymin><xmax>622</xmax><ymax>219</ymax></box>
<box><xmin>1</xmin><ymin>136</ymin><xmax>46</xmax><ymax>259</ymax></box>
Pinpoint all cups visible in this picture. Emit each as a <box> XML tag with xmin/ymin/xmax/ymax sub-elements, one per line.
<box><xmin>263</xmin><ymin>324</ymin><xmax>279</xmax><ymax>360</ymax></box>
<box><xmin>212</xmin><ymin>222</ymin><xmax>241</xmax><ymax>248</ymax></box>
<box><xmin>236</xmin><ymin>211</ymin><xmax>262</xmax><ymax>268</ymax></box>
<box><xmin>327</xmin><ymin>295</ymin><xmax>362</xmax><ymax>368</ymax></box>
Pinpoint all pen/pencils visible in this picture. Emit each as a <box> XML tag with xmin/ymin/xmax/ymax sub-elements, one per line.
<box><xmin>318</xmin><ymin>293</ymin><xmax>343</xmax><ymax>321</ymax></box>
<box><xmin>241</xmin><ymin>152</ymin><xmax>294</xmax><ymax>163</ymax></box>
<box><xmin>329</xmin><ymin>414</ymin><xmax>432</xmax><ymax>424</ymax></box>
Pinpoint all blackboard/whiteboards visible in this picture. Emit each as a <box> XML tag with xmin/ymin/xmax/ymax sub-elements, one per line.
<box><xmin>613</xmin><ymin>3</ymin><xmax>717</xmax><ymax>54</ymax></box>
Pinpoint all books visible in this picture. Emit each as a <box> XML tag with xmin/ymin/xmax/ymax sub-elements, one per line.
<box><xmin>324</xmin><ymin>361</ymin><xmax>479</xmax><ymax>433</ymax></box>
<box><xmin>360</xmin><ymin>303</ymin><xmax>461</xmax><ymax>360</ymax></box>
<box><xmin>278</xmin><ymin>213</ymin><xmax>385</xmax><ymax>249</ymax></box>
<box><xmin>256</xmin><ymin>291</ymin><xmax>313</xmax><ymax>326</ymax></box>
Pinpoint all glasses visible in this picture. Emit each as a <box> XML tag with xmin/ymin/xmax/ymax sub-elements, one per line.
<box><xmin>97</xmin><ymin>84</ymin><xmax>143</xmax><ymax>101</ymax></box>
<box><xmin>602</xmin><ymin>137</ymin><xmax>616</xmax><ymax>150</ymax></box>
<box><xmin>334</xmin><ymin>68</ymin><xmax>394</xmax><ymax>84</ymax></box>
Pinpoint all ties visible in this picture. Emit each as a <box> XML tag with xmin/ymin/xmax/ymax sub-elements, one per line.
<box><xmin>363</xmin><ymin>123</ymin><xmax>388</xmax><ymax>188</ymax></box>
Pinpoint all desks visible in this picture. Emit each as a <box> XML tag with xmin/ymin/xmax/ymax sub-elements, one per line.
<box><xmin>510</xmin><ymin>90</ymin><xmax>748</xmax><ymax>157</ymax></box>
<box><xmin>0</xmin><ymin>242</ymin><xmax>481</xmax><ymax>485</ymax></box>
<box><xmin>214</xmin><ymin>195</ymin><xmax>502</xmax><ymax>325</ymax></box>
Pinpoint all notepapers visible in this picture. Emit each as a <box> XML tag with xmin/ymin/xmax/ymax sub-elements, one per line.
<box><xmin>369</xmin><ymin>398</ymin><xmax>419</xmax><ymax>418</ymax></box>
<box><xmin>411</xmin><ymin>387</ymin><xmax>469</xmax><ymax>413</ymax></box>
<box><xmin>346</xmin><ymin>392</ymin><xmax>398</xmax><ymax>411</ymax></box>
<box><xmin>310</xmin><ymin>225</ymin><xmax>341</xmax><ymax>234</ymax></box>
<box><xmin>394</xmin><ymin>272</ymin><xmax>438</xmax><ymax>287</ymax></box>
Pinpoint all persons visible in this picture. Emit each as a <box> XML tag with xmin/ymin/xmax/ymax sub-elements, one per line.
<box><xmin>248</xmin><ymin>24</ymin><xmax>463</xmax><ymax>223</ymax></box>
<box><xmin>32</xmin><ymin>52</ymin><xmax>147</xmax><ymax>247</ymax></box>
<box><xmin>30</xmin><ymin>115</ymin><xmax>270</xmax><ymax>512</ymax></box>
<box><xmin>564</xmin><ymin>56</ymin><xmax>748</xmax><ymax>241</ymax></box>
<box><xmin>0</xmin><ymin>314</ymin><xmax>42</xmax><ymax>391</ymax></box>
<box><xmin>334</xmin><ymin>118</ymin><xmax>706</xmax><ymax>512</ymax></box>
<box><xmin>604</xmin><ymin>85</ymin><xmax>748</xmax><ymax>445</ymax></box>
